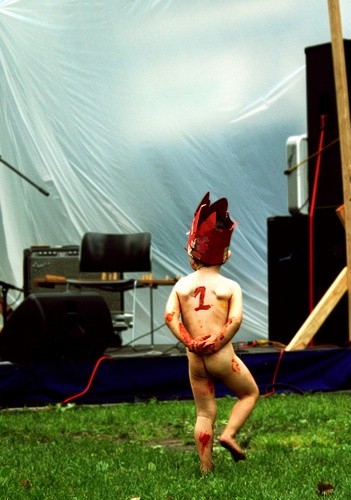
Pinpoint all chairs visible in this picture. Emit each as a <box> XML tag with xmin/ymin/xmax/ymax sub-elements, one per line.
<box><xmin>66</xmin><ymin>232</ymin><xmax>154</xmax><ymax>351</ymax></box>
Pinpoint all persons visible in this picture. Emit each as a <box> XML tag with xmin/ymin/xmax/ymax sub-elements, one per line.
<box><xmin>163</xmin><ymin>191</ymin><xmax>261</xmax><ymax>478</ymax></box>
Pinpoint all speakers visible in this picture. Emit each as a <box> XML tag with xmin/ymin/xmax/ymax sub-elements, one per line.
<box><xmin>267</xmin><ymin>215</ymin><xmax>313</xmax><ymax>344</ymax></box>
<box><xmin>283</xmin><ymin>134</ymin><xmax>308</xmax><ymax>214</ymax></box>
<box><xmin>22</xmin><ymin>245</ymin><xmax>122</xmax><ymax>317</ymax></box>
<box><xmin>0</xmin><ymin>293</ymin><xmax>114</xmax><ymax>359</ymax></box>
